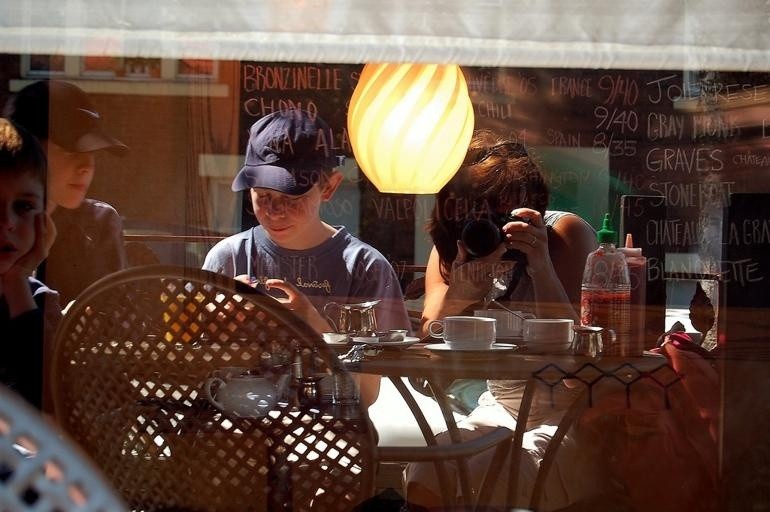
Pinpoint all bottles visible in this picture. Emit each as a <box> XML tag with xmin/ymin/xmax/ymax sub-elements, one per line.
<box><xmin>613</xmin><ymin>232</ymin><xmax>649</xmax><ymax>359</ymax></box>
<box><xmin>293</xmin><ymin>344</ymin><xmax>306</xmax><ymax>385</ymax></box>
<box><xmin>309</xmin><ymin>343</ymin><xmax>332</xmax><ymax>404</ymax></box>
<box><xmin>577</xmin><ymin>212</ymin><xmax>630</xmax><ymax>357</ymax></box>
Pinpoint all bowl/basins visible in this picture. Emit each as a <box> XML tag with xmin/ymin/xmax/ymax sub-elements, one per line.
<box><xmin>377</xmin><ymin>329</ymin><xmax>408</xmax><ymax>341</ymax></box>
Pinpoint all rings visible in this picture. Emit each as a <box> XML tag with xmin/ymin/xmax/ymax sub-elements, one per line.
<box><xmin>530</xmin><ymin>237</ymin><xmax>536</xmax><ymax>245</ymax></box>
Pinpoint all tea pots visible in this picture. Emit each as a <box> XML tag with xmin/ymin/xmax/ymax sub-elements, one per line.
<box><xmin>206</xmin><ymin>373</ymin><xmax>290</xmax><ymax>423</ymax></box>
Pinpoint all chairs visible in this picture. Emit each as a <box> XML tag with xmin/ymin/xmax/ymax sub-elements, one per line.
<box><xmin>659</xmin><ymin>333</ymin><xmax>720</xmax><ymax>443</ymax></box>
<box><xmin>46</xmin><ymin>263</ymin><xmax>513</xmax><ymax>510</ymax></box>
<box><xmin>0</xmin><ymin>384</ymin><xmax>129</xmax><ymax>511</ymax></box>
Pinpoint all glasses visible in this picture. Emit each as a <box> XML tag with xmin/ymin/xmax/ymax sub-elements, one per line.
<box><xmin>460</xmin><ymin>142</ymin><xmax>529</xmax><ymax>167</ymax></box>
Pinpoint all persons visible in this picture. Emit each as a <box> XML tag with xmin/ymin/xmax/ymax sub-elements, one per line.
<box><xmin>167</xmin><ymin>107</ymin><xmax>415</xmax><ymax>512</ymax></box>
<box><xmin>10</xmin><ymin>77</ymin><xmax>129</xmax><ymax>310</ymax></box>
<box><xmin>0</xmin><ymin>117</ymin><xmax>64</xmax><ymax>411</ymax></box>
<box><xmin>402</xmin><ymin>128</ymin><xmax>609</xmax><ymax>512</ymax></box>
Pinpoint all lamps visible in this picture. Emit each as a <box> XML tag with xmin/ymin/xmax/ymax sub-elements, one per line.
<box><xmin>346</xmin><ymin>65</ymin><xmax>474</xmax><ymax>194</ymax></box>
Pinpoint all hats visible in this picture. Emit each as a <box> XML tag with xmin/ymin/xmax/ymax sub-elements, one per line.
<box><xmin>5</xmin><ymin>79</ymin><xmax>128</xmax><ymax>160</ymax></box>
<box><xmin>231</xmin><ymin>108</ymin><xmax>343</xmax><ymax>197</ymax></box>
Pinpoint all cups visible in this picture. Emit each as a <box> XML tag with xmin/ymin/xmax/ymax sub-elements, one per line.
<box><xmin>295</xmin><ymin>375</ymin><xmax>325</xmax><ymax>412</ymax></box>
<box><xmin>323</xmin><ymin>299</ymin><xmax>382</xmax><ymax>339</ymax></box>
<box><xmin>332</xmin><ymin>371</ymin><xmax>361</xmax><ymax>407</ymax></box>
<box><xmin>159</xmin><ymin>290</ymin><xmax>206</xmax><ymax>349</ymax></box>
<box><xmin>572</xmin><ymin>324</ymin><xmax>618</xmax><ymax>359</ymax></box>
<box><xmin>427</xmin><ymin>315</ymin><xmax>498</xmax><ymax>350</ymax></box>
<box><xmin>473</xmin><ymin>309</ymin><xmax>538</xmax><ymax>339</ymax></box>
<box><xmin>522</xmin><ymin>318</ymin><xmax>575</xmax><ymax>353</ymax></box>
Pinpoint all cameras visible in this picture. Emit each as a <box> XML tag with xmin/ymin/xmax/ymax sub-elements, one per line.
<box><xmin>430</xmin><ymin>213</ymin><xmax>531</xmax><ymax>264</ymax></box>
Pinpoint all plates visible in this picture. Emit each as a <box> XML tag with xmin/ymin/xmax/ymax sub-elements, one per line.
<box><xmin>489</xmin><ymin>337</ymin><xmax>534</xmax><ymax>344</ymax></box>
<box><xmin>351</xmin><ymin>336</ymin><xmax>419</xmax><ymax>348</ymax></box>
<box><xmin>424</xmin><ymin>343</ymin><xmax>517</xmax><ymax>354</ymax></box>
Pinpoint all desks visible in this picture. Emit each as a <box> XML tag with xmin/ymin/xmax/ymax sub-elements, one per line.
<box><xmin>302</xmin><ymin>335</ymin><xmax>671</xmax><ymax>511</ymax></box>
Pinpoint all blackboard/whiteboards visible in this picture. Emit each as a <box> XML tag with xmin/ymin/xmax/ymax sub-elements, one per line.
<box><xmin>239</xmin><ymin>62</ymin><xmax>770</xmax><ymax>310</ymax></box>
